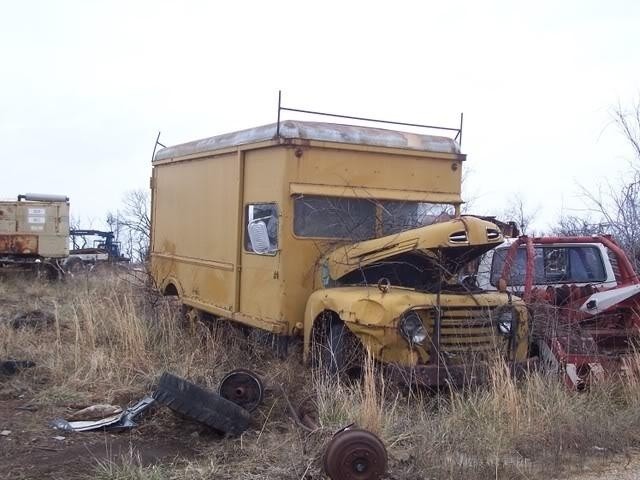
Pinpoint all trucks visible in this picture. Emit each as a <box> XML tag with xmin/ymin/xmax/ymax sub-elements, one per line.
<box><xmin>1</xmin><ymin>193</ymin><xmax>128</xmax><ymax>288</ymax></box>
<box><xmin>148</xmin><ymin>90</ymin><xmax>532</xmax><ymax>406</ymax></box>
<box><xmin>464</xmin><ymin>223</ymin><xmax>638</xmax><ymax>400</ymax></box>
